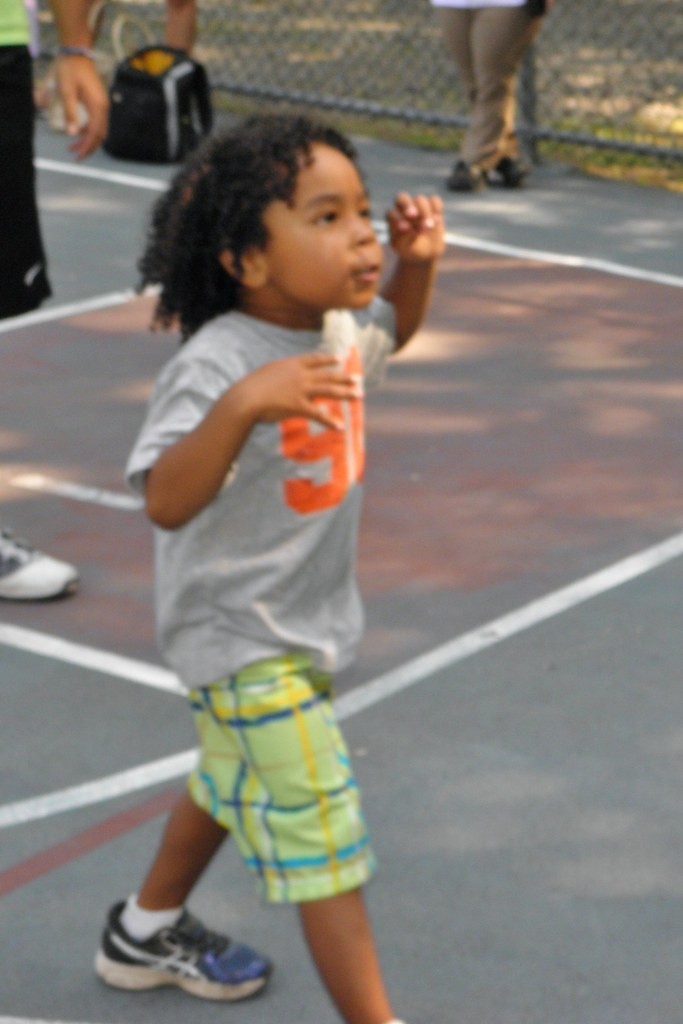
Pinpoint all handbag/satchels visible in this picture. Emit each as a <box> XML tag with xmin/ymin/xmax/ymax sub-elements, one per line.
<box><xmin>102</xmin><ymin>15</ymin><xmax>214</xmax><ymax>162</ymax></box>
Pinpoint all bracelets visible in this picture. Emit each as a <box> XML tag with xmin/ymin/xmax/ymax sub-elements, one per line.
<box><xmin>57</xmin><ymin>45</ymin><xmax>100</xmax><ymax>61</ymax></box>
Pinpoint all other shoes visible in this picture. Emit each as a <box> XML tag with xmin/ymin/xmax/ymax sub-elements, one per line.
<box><xmin>447</xmin><ymin>160</ymin><xmax>489</xmax><ymax>193</ymax></box>
<box><xmin>496</xmin><ymin>159</ymin><xmax>528</xmax><ymax>184</ymax></box>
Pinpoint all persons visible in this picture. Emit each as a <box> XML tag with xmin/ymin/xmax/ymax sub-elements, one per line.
<box><xmin>121</xmin><ymin>114</ymin><xmax>447</xmax><ymax>1024</ymax></box>
<box><xmin>28</xmin><ymin>0</ymin><xmax>552</xmax><ymax>193</ymax></box>
<box><xmin>0</xmin><ymin>1</ymin><xmax>109</xmax><ymax>603</ymax></box>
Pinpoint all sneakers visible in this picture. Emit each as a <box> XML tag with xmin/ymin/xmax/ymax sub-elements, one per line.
<box><xmin>94</xmin><ymin>900</ymin><xmax>273</xmax><ymax>1001</ymax></box>
<box><xmin>0</xmin><ymin>527</ymin><xmax>82</xmax><ymax>603</ymax></box>
